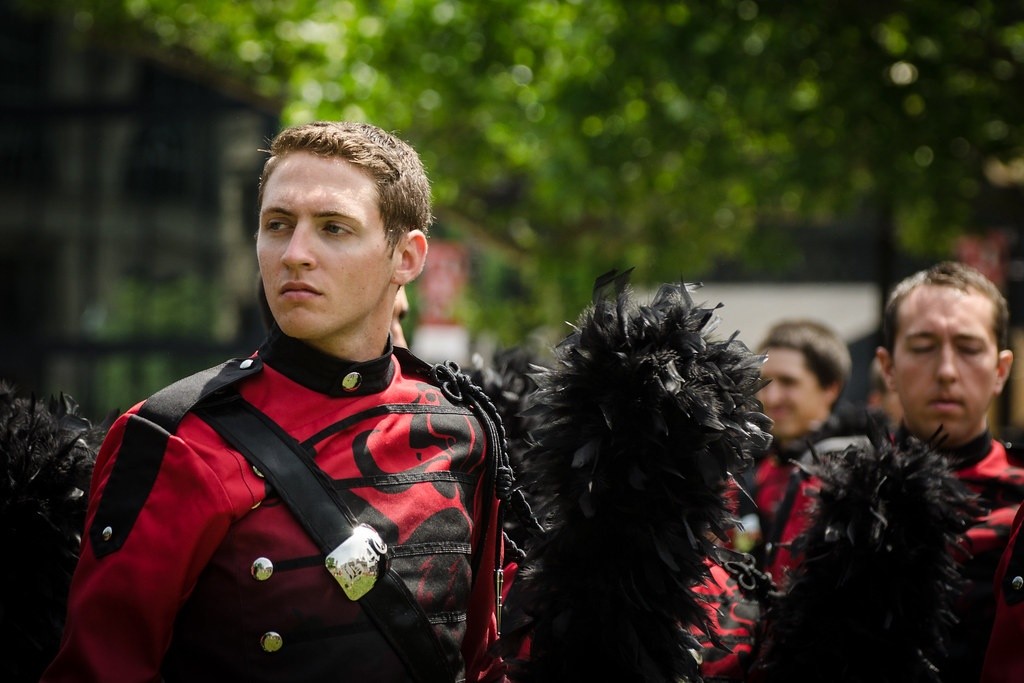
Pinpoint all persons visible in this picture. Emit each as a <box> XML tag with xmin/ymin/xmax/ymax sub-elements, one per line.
<box><xmin>42</xmin><ymin>118</ymin><xmax>510</xmax><ymax>676</ymax></box>
<box><xmin>493</xmin><ymin>265</ymin><xmax>1022</xmax><ymax>683</ymax></box>
